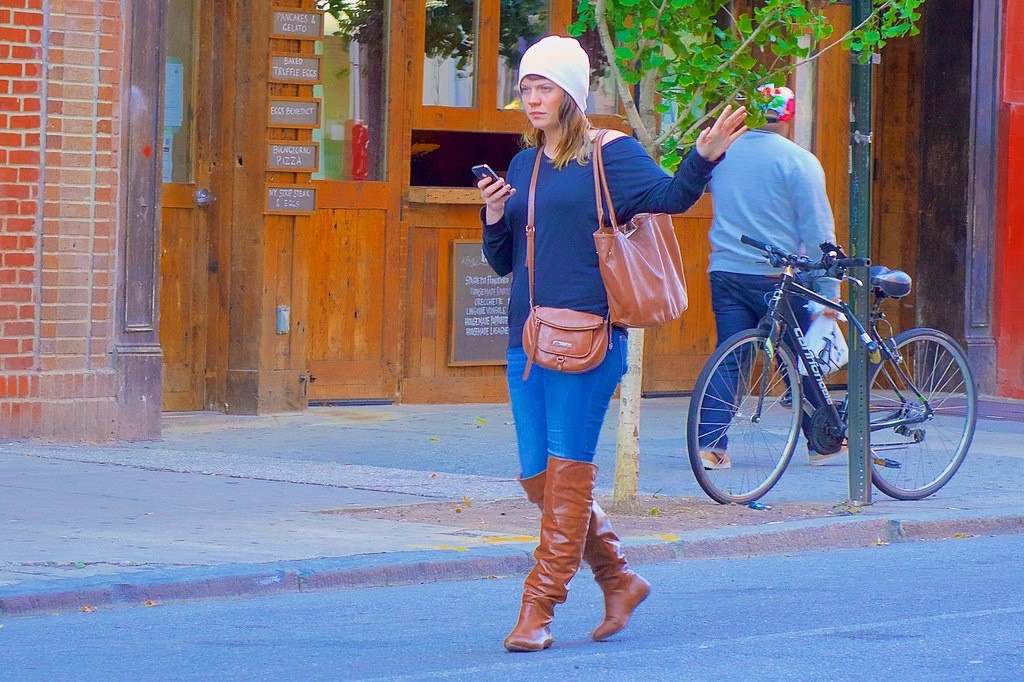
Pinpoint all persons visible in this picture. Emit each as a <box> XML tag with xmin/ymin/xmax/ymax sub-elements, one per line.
<box><xmin>693</xmin><ymin>85</ymin><xmax>849</xmax><ymax>470</ymax></box>
<box><xmin>478</xmin><ymin>37</ymin><xmax>750</xmax><ymax>650</ymax></box>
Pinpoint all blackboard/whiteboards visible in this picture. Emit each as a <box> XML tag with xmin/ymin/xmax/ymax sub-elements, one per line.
<box><xmin>265</xmin><ymin>138</ymin><xmax>320</xmax><ymax>174</ymax></box>
<box><xmin>267</xmin><ymin>95</ymin><xmax>323</xmax><ymax>130</ymax></box>
<box><xmin>268</xmin><ymin>50</ymin><xmax>325</xmax><ymax>86</ymax></box>
<box><xmin>263</xmin><ymin>181</ymin><xmax>318</xmax><ymax>216</ymax></box>
<box><xmin>447</xmin><ymin>236</ymin><xmax>515</xmax><ymax>369</ymax></box>
<box><xmin>270</xmin><ymin>6</ymin><xmax>325</xmax><ymax>42</ymax></box>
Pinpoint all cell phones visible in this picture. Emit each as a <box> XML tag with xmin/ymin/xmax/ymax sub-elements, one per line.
<box><xmin>472</xmin><ymin>164</ymin><xmax>511</xmax><ymax>197</ymax></box>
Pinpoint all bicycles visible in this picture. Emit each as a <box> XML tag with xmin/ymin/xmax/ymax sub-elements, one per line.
<box><xmin>685</xmin><ymin>233</ymin><xmax>979</xmax><ymax>507</ymax></box>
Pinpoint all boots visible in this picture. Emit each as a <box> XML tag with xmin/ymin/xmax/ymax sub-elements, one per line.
<box><xmin>504</xmin><ymin>457</ymin><xmax>600</xmax><ymax>653</ymax></box>
<box><xmin>517</xmin><ymin>469</ymin><xmax>653</xmax><ymax>641</ymax></box>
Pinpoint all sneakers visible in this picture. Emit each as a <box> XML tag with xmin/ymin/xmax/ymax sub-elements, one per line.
<box><xmin>810</xmin><ymin>437</ymin><xmax>850</xmax><ymax>464</ymax></box>
<box><xmin>691</xmin><ymin>446</ymin><xmax>733</xmax><ymax>469</ymax></box>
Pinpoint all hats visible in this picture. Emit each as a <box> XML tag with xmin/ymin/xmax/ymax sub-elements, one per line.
<box><xmin>753</xmin><ymin>85</ymin><xmax>798</xmax><ymax>124</ymax></box>
<box><xmin>518</xmin><ymin>36</ymin><xmax>589</xmax><ymax>114</ymax></box>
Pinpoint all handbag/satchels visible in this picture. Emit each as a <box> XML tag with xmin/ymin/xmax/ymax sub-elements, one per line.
<box><xmin>593</xmin><ymin>132</ymin><xmax>691</xmax><ymax>326</ymax></box>
<box><xmin>522</xmin><ymin>303</ymin><xmax>612</xmax><ymax>374</ymax></box>
<box><xmin>798</xmin><ymin>305</ymin><xmax>849</xmax><ymax>377</ymax></box>
